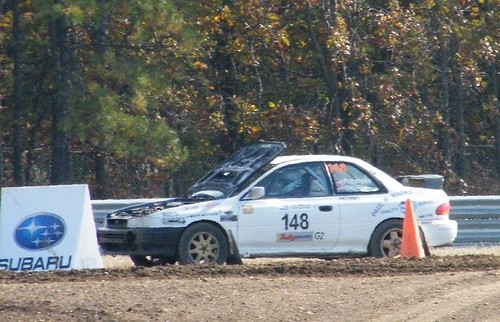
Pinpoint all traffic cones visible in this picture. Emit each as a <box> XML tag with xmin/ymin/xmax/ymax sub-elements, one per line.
<box><xmin>400</xmin><ymin>198</ymin><xmax>426</xmax><ymax>260</ymax></box>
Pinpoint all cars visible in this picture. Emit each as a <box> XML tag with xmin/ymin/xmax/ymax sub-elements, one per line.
<box><xmin>95</xmin><ymin>140</ymin><xmax>459</xmax><ymax>266</ymax></box>
